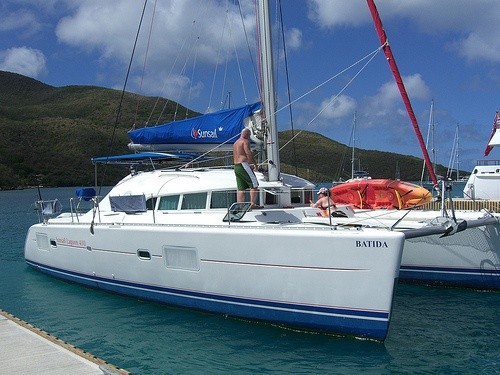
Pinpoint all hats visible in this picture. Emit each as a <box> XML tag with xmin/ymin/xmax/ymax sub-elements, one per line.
<box><xmin>317</xmin><ymin>187</ymin><xmax>329</xmax><ymax>194</ymax></box>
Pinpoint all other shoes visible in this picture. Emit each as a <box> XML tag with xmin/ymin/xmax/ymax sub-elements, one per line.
<box><xmin>251</xmin><ymin>204</ymin><xmax>264</xmax><ymax>209</ymax></box>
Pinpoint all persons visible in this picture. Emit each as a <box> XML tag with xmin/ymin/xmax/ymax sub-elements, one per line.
<box><xmin>309</xmin><ymin>187</ymin><xmax>348</xmax><ymax>218</ymax></box>
<box><xmin>233</xmin><ymin>128</ymin><xmax>264</xmax><ymax>209</ymax></box>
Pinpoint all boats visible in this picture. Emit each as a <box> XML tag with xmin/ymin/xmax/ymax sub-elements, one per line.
<box><xmin>24</xmin><ymin>0</ymin><xmax>500</xmax><ymax>341</ymax></box>
